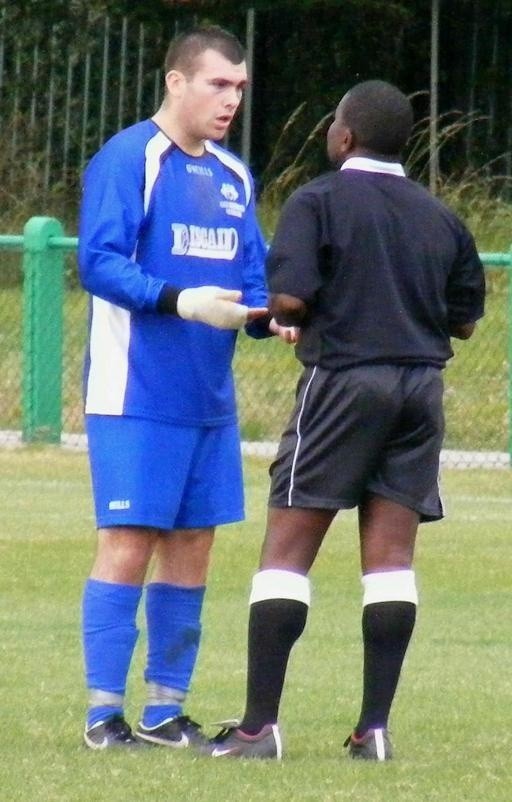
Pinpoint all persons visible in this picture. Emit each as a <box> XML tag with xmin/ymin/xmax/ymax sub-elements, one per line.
<box><xmin>74</xmin><ymin>24</ymin><xmax>303</xmax><ymax>753</ymax></box>
<box><xmin>196</xmin><ymin>77</ymin><xmax>487</xmax><ymax>765</ymax></box>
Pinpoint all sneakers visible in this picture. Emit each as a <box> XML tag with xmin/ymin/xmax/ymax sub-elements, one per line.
<box><xmin>344</xmin><ymin>725</ymin><xmax>391</xmax><ymax>759</ymax></box>
<box><xmin>201</xmin><ymin>722</ymin><xmax>282</xmax><ymax>760</ymax></box>
<box><xmin>135</xmin><ymin>714</ymin><xmax>212</xmax><ymax>751</ymax></box>
<box><xmin>83</xmin><ymin>716</ymin><xmax>144</xmax><ymax>751</ymax></box>
